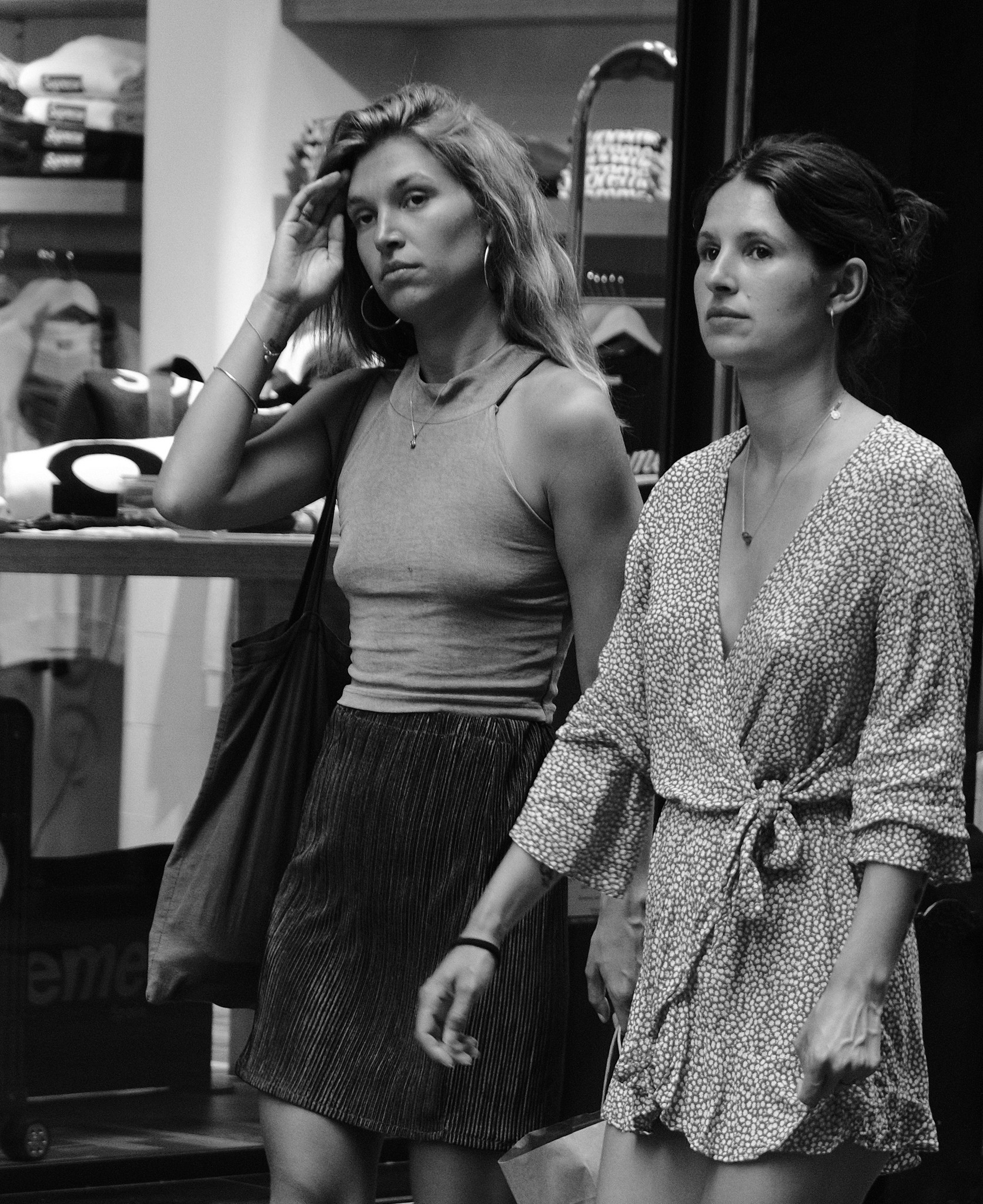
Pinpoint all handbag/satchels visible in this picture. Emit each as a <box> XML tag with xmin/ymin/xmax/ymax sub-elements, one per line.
<box><xmin>143</xmin><ymin>618</ymin><xmax>351</xmax><ymax>1003</ymax></box>
<box><xmin>497</xmin><ymin>1024</ymin><xmax>624</xmax><ymax>1204</ymax></box>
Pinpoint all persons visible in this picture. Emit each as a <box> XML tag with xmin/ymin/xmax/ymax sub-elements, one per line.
<box><xmin>157</xmin><ymin>79</ymin><xmax>642</xmax><ymax>1203</ymax></box>
<box><xmin>410</xmin><ymin>135</ymin><xmax>977</xmax><ymax>1203</ymax></box>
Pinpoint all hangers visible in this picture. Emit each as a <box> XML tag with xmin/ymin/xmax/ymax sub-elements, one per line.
<box><xmin>0</xmin><ymin>241</ymin><xmax>140</xmax><ymax>358</ymax></box>
<box><xmin>573</xmin><ymin>269</ymin><xmax>669</xmax><ymax>359</ymax></box>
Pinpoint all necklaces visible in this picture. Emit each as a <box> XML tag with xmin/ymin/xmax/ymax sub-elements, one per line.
<box><xmin>740</xmin><ymin>392</ymin><xmax>846</xmax><ymax>546</ymax></box>
<box><xmin>409</xmin><ymin>331</ymin><xmax>514</xmax><ymax>450</ymax></box>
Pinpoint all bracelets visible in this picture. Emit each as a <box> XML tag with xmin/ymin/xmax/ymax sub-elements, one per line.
<box><xmin>243</xmin><ymin>315</ymin><xmax>283</xmax><ymax>366</ymax></box>
<box><xmin>215</xmin><ymin>366</ymin><xmax>257</xmax><ymax>416</ymax></box>
<box><xmin>451</xmin><ymin>936</ymin><xmax>501</xmax><ymax>966</ymax></box>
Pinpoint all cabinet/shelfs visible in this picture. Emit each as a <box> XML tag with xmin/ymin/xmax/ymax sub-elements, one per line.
<box><xmin>0</xmin><ymin>0</ymin><xmax>677</xmax><ymax>245</ymax></box>
